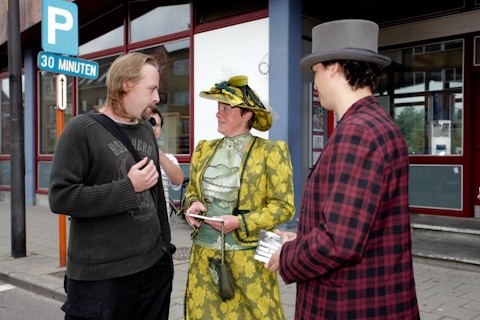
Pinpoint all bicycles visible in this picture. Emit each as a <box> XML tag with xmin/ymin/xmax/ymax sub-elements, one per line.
<box><xmin>166</xmin><ymin>177</ymin><xmax>189</xmax><ymax>220</ymax></box>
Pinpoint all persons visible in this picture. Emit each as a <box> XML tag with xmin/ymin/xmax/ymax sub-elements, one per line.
<box><xmin>182</xmin><ymin>75</ymin><xmax>296</xmax><ymax>320</ymax></box>
<box><xmin>141</xmin><ymin>105</ymin><xmax>185</xmax><ymax>224</ymax></box>
<box><xmin>46</xmin><ymin>52</ymin><xmax>176</xmax><ymax>319</ymax></box>
<box><xmin>263</xmin><ymin>18</ymin><xmax>421</xmax><ymax>320</ymax></box>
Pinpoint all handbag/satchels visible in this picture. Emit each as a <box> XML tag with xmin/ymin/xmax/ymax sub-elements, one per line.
<box><xmin>207</xmin><ymin>222</ymin><xmax>237</xmax><ymax>303</ymax></box>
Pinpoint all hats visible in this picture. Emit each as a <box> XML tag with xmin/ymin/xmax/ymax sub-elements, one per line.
<box><xmin>300</xmin><ymin>18</ymin><xmax>392</xmax><ymax>80</ymax></box>
<box><xmin>198</xmin><ymin>76</ymin><xmax>273</xmax><ymax>132</ymax></box>
